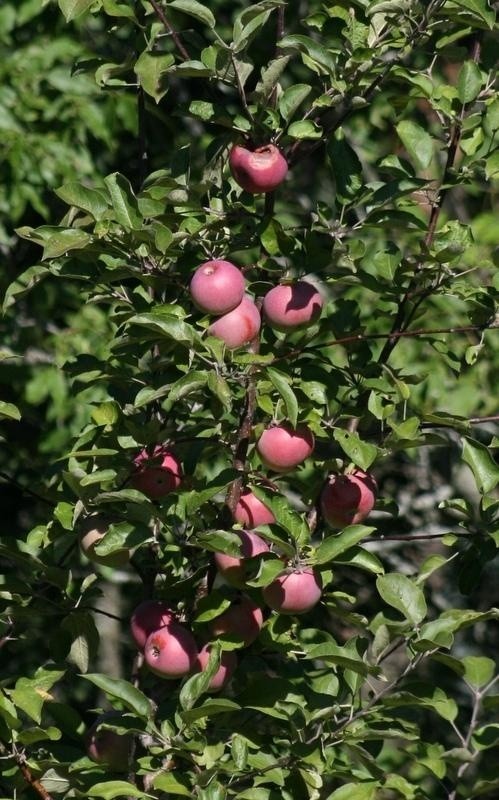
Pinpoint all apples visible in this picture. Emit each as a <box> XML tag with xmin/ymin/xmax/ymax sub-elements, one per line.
<box><xmin>207</xmin><ymin>296</ymin><xmax>259</xmax><ymax>350</ymax></box>
<box><xmin>86</xmin><ymin>725</ymin><xmax>137</xmax><ymax>772</ymax></box>
<box><xmin>263</xmin><ymin>566</ymin><xmax>323</xmax><ymax>616</ymax></box>
<box><xmin>229</xmin><ymin>476</ymin><xmax>275</xmax><ymax>528</ymax></box>
<box><xmin>143</xmin><ymin>623</ymin><xmax>197</xmax><ymax>678</ymax></box>
<box><xmin>320</xmin><ymin>467</ymin><xmax>374</xmax><ymax>529</ymax></box>
<box><xmin>261</xmin><ymin>280</ymin><xmax>323</xmax><ymax>331</ymax></box>
<box><xmin>130</xmin><ymin>444</ymin><xmax>182</xmax><ymax>499</ymax></box>
<box><xmin>196</xmin><ymin>642</ymin><xmax>236</xmax><ymax>691</ymax></box>
<box><xmin>191</xmin><ymin>261</ymin><xmax>245</xmax><ymax>316</ymax></box>
<box><xmin>255</xmin><ymin>426</ymin><xmax>315</xmax><ymax>471</ymax></box>
<box><xmin>228</xmin><ymin>143</ymin><xmax>286</xmax><ymax>192</ymax></box>
<box><xmin>209</xmin><ymin>594</ymin><xmax>263</xmax><ymax>649</ymax></box>
<box><xmin>213</xmin><ymin>531</ymin><xmax>270</xmax><ymax>592</ymax></box>
<box><xmin>131</xmin><ymin>602</ymin><xmax>182</xmax><ymax>649</ymax></box>
